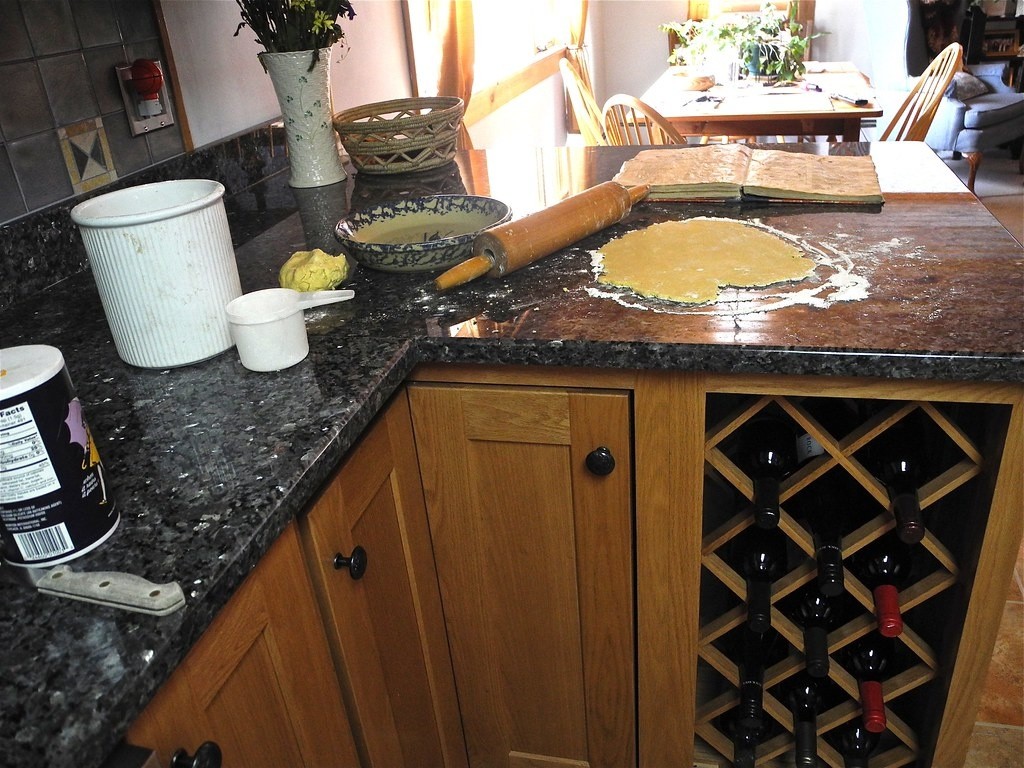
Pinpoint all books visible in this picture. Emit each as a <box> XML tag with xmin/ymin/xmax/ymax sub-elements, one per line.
<box><xmin>613</xmin><ymin>143</ymin><xmax>886</xmax><ymax>204</ymax></box>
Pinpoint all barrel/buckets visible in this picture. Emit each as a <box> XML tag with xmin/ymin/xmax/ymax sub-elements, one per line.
<box><xmin>70</xmin><ymin>178</ymin><xmax>243</xmax><ymax>371</ymax></box>
<box><xmin>0</xmin><ymin>345</ymin><xmax>121</xmax><ymax>568</ymax></box>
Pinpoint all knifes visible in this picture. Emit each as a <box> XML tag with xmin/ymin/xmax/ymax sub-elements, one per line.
<box><xmin>1</xmin><ymin>563</ymin><xmax>185</xmax><ymax>616</ymax></box>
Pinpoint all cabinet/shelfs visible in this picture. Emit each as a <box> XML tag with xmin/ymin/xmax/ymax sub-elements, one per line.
<box><xmin>412</xmin><ymin>384</ymin><xmax>656</xmax><ymax>764</ymax></box>
<box><xmin>643</xmin><ymin>385</ymin><xmax>1024</xmax><ymax>767</ymax></box>
<box><xmin>132</xmin><ymin>387</ymin><xmax>467</xmax><ymax>766</ymax></box>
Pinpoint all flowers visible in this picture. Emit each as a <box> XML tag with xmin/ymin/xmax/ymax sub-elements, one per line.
<box><xmin>234</xmin><ymin>1</ymin><xmax>355</xmax><ymax>61</ymax></box>
<box><xmin>667</xmin><ymin>3</ymin><xmax>805</xmax><ymax>84</ymax></box>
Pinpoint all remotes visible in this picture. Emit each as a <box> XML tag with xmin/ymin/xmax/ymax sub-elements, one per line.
<box><xmin>832</xmin><ymin>93</ymin><xmax>868</xmax><ymax>106</ymax></box>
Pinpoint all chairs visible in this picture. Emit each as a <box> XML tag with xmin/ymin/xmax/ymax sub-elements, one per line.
<box><xmin>558</xmin><ymin>58</ymin><xmax>620</xmax><ymax>147</ymax></box>
<box><xmin>929</xmin><ymin>62</ymin><xmax>1022</xmax><ymax>191</ymax></box>
<box><xmin>601</xmin><ymin>93</ymin><xmax>686</xmax><ymax>148</ymax></box>
<box><xmin>881</xmin><ymin>42</ymin><xmax>964</xmax><ymax>142</ymax></box>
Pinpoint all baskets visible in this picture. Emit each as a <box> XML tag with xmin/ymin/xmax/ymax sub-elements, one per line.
<box><xmin>331</xmin><ymin>96</ymin><xmax>466</xmax><ymax>175</ymax></box>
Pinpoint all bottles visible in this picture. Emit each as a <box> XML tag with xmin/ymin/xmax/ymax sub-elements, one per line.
<box><xmin>729</xmin><ymin>396</ymin><xmax>927</xmax><ymax>768</ymax></box>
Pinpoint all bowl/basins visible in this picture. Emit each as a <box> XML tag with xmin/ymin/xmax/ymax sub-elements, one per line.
<box><xmin>333</xmin><ymin>194</ymin><xmax>513</xmax><ymax>274</ymax></box>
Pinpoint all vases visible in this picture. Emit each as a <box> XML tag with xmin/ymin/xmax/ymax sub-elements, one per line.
<box><xmin>679</xmin><ymin>42</ymin><xmax>740</xmax><ymax>81</ymax></box>
<box><xmin>255</xmin><ymin>44</ymin><xmax>347</xmax><ymax>191</ymax></box>
<box><xmin>740</xmin><ymin>44</ymin><xmax>794</xmax><ymax>82</ymax></box>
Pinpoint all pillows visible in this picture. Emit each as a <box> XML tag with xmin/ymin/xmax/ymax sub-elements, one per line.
<box><xmin>951</xmin><ymin>70</ymin><xmax>988</xmax><ymax>100</ymax></box>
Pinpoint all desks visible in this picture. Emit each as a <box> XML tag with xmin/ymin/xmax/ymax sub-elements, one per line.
<box><xmin>630</xmin><ymin>62</ymin><xmax>885</xmax><ymax>144</ymax></box>
